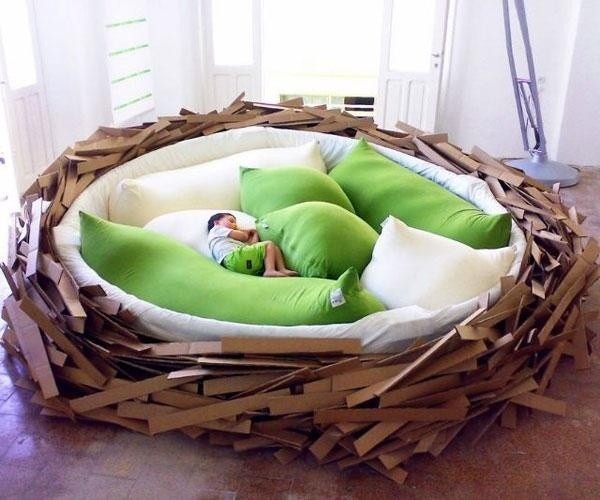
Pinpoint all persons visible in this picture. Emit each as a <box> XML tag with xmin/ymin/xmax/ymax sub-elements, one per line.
<box><xmin>207</xmin><ymin>213</ymin><xmax>299</xmax><ymax>277</ymax></box>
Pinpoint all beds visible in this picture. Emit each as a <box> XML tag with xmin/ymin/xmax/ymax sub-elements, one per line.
<box><xmin>0</xmin><ymin>91</ymin><xmax>600</xmax><ymax>484</ymax></box>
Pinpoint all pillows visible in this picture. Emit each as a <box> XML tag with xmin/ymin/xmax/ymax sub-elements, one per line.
<box><xmin>359</xmin><ymin>217</ymin><xmax>513</xmax><ymax>311</ymax></box>
<box><xmin>324</xmin><ymin>136</ymin><xmax>513</xmax><ymax>250</ymax></box>
<box><xmin>255</xmin><ymin>203</ymin><xmax>380</xmax><ymax>284</ymax></box>
<box><xmin>235</xmin><ymin>165</ymin><xmax>355</xmax><ymax>215</ymax></box>
<box><xmin>79</xmin><ymin>207</ymin><xmax>385</xmax><ymax>327</ymax></box>
<box><xmin>106</xmin><ymin>141</ymin><xmax>324</xmax><ymax>228</ymax></box>
<box><xmin>143</xmin><ymin>207</ymin><xmax>257</xmax><ymax>260</ymax></box>
